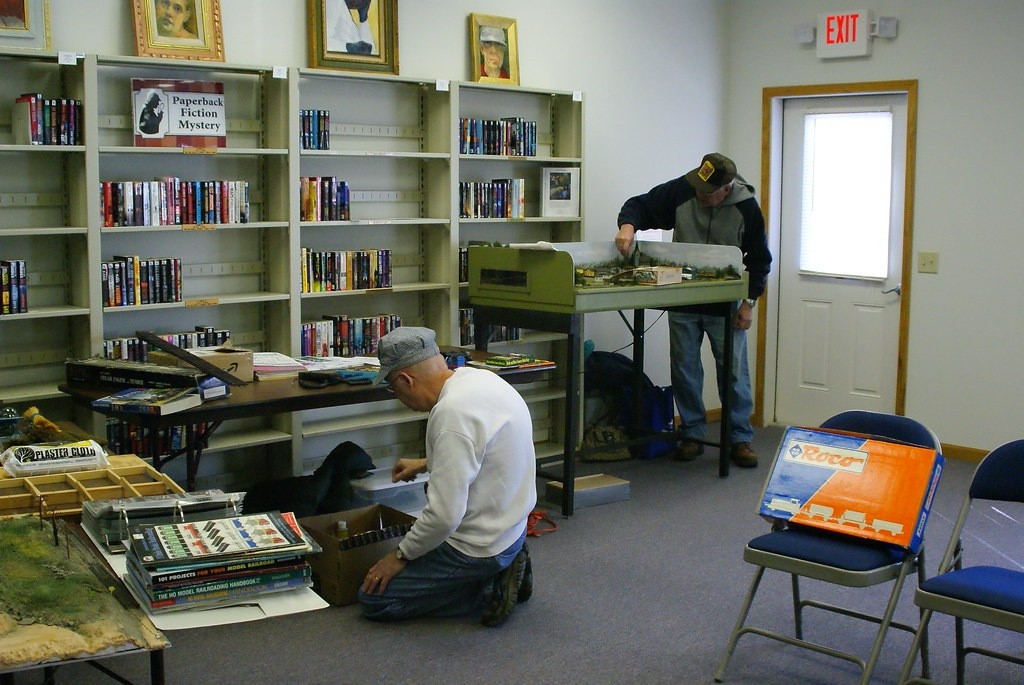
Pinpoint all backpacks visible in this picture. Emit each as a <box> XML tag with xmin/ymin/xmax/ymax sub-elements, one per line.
<box><xmin>584</xmin><ymin>349</ymin><xmax>684</xmax><ymax>460</ymax></box>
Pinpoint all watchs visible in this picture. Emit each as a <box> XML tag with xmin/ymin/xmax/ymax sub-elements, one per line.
<box><xmin>397</xmin><ymin>546</ymin><xmax>407</xmax><ymax>561</ymax></box>
<box><xmin>742</xmin><ymin>299</ymin><xmax>755</xmax><ymax>307</ymax></box>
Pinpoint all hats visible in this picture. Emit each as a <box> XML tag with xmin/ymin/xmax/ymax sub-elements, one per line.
<box><xmin>372</xmin><ymin>327</ymin><xmax>439</xmax><ymax>387</ymax></box>
<box><xmin>685</xmin><ymin>153</ymin><xmax>737</xmax><ymax>192</ymax></box>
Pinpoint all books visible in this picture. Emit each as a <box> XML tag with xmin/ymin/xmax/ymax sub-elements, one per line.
<box><xmin>104</xmin><ymin>324</ymin><xmax>230</xmax><ymax>363</ymax></box>
<box><xmin>300</xmin><ymin>176</ymin><xmax>352</xmax><ymax>221</ymax></box>
<box><xmin>300</xmin><ymin>247</ymin><xmax>392</xmax><ymax>293</ymax></box>
<box><xmin>10</xmin><ymin>92</ymin><xmax>82</xmax><ymax>145</ymax></box>
<box><xmin>299</xmin><ymin>109</ymin><xmax>330</xmax><ymax>150</ymax></box>
<box><xmin>0</xmin><ymin>260</ymin><xmax>29</xmax><ymax>314</ymax></box>
<box><xmin>539</xmin><ymin>166</ymin><xmax>579</xmax><ymax>217</ymax></box>
<box><xmin>92</xmin><ymin>386</ymin><xmax>202</xmax><ymax>416</ymax></box>
<box><xmin>460</xmin><ymin>117</ymin><xmax>537</xmax><ymax>156</ymax></box>
<box><xmin>101</xmin><ymin>256</ymin><xmax>182</xmax><ymax>307</ymax></box>
<box><xmin>100</xmin><ymin>177</ymin><xmax>250</xmax><ymax>227</ymax></box>
<box><xmin>80</xmin><ymin>490</ymin><xmax>322</xmax><ymax>614</ymax></box>
<box><xmin>106</xmin><ymin>417</ymin><xmax>208</xmax><ymax>457</ymax></box>
<box><xmin>459</xmin><ymin>179</ymin><xmax>525</xmax><ymax>218</ymax></box>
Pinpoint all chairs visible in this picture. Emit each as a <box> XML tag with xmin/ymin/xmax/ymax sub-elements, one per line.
<box><xmin>711</xmin><ymin>409</ymin><xmax>942</xmax><ymax>685</ymax></box>
<box><xmin>899</xmin><ymin>437</ymin><xmax>1024</xmax><ymax>685</ymax></box>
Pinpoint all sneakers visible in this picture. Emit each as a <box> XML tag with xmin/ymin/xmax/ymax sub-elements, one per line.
<box><xmin>516</xmin><ymin>542</ymin><xmax>533</xmax><ymax>603</ymax></box>
<box><xmin>480</xmin><ymin>560</ymin><xmax>517</xmax><ymax>626</ymax></box>
<box><xmin>730</xmin><ymin>441</ymin><xmax>758</xmax><ymax>468</ymax></box>
<box><xmin>675</xmin><ymin>439</ymin><xmax>705</xmax><ymax>461</ymax></box>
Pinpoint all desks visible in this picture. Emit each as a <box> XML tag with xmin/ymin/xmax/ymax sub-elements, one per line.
<box><xmin>0</xmin><ymin>507</ymin><xmax>172</xmax><ymax>685</ymax></box>
<box><xmin>466</xmin><ymin>237</ymin><xmax>751</xmax><ymax>518</ymax></box>
<box><xmin>58</xmin><ymin>345</ymin><xmax>555</xmax><ymax>488</ymax></box>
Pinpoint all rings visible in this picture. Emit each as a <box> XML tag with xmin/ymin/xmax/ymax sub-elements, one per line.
<box><xmin>375</xmin><ymin>577</ymin><xmax>379</xmax><ymax>581</ymax></box>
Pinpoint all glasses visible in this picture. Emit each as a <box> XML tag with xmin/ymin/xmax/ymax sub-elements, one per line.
<box><xmin>385</xmin><ymin>374</ymin><xmax>414</xmax><ymax>394</ymax></box>
<box><xmin>705</xmin><ymin>193</ymin><xmax>712</xmax><ymax>198</ymax></box>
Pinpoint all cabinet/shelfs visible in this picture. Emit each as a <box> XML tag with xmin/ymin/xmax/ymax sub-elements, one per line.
<box><xmin>91</xmin><ymin>46</ymin><xmax>296</xmax><ymax>488</ymax></box>
<box><xmin>291</xmin><ymin>64</ymin><xmax>457</xmax><ymax>485</ymax></box>
<box><xmin>454</xmin><ymin>77</ymin><xmax>593</xmax><ymax>467</ymax></box>
<box><xmin>0</xmin><ymin>50</ymin><xmax>93</xmax><ymax>451</ymax></box>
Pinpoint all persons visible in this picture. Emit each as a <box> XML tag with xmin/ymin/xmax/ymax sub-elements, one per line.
<box><xmin>358</xmin><ymin>327</ymin><xmax>538</xmax><ymax>628</ymax></box>
<box><xmin>616</xmin><ymin>153</ymin><xmax>774</xmax><ymax>468</ymax></box>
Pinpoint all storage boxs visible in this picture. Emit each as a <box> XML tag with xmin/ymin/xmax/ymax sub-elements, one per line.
<box><xmin>349</xmin><ymin>464</ymin><xmax>430</xmax><ymax>512</ymax></box>
<box><xmin>147</xmin><ymin>345</ymin><xmax>254</xmax><ymax>382</ymax></box>
<box><xmin>755</xmin><ymin>424</ymin><xmax>946</xmax><ymax>554</ymax></box>
<box><xmin>65</xmin><ymin>352</ymin><xmax>217</xmax><ymax>396</ymax></box>
<box><xmin>545</xmin><ymin>472</ymin><xmax>633</xmax><ymax>509</ymax></box>
<box><xmin>296</xmin><ymin>502</ymin><xmax>419</xmax><ymax>609</ymax></box>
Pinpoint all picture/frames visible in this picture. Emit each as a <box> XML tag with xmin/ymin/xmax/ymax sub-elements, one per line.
<box><xmin>0</xmin><ymin>0</ymin><xmax>53</xmax><ymax>53</ymax></box>
<box><xmin>129</xmin><ymin>0</ymin><xmax>226</xmax><ymax>63</ymax></box>
<box><xmin>305</xmin><ymin>0</ymin><xmax>401</xmax><ymax>76</ymax></box>
<box><xmin>469</xmin><ymin>11</ymin><xmax>520</xmax><ymax>87</ymax></box>
